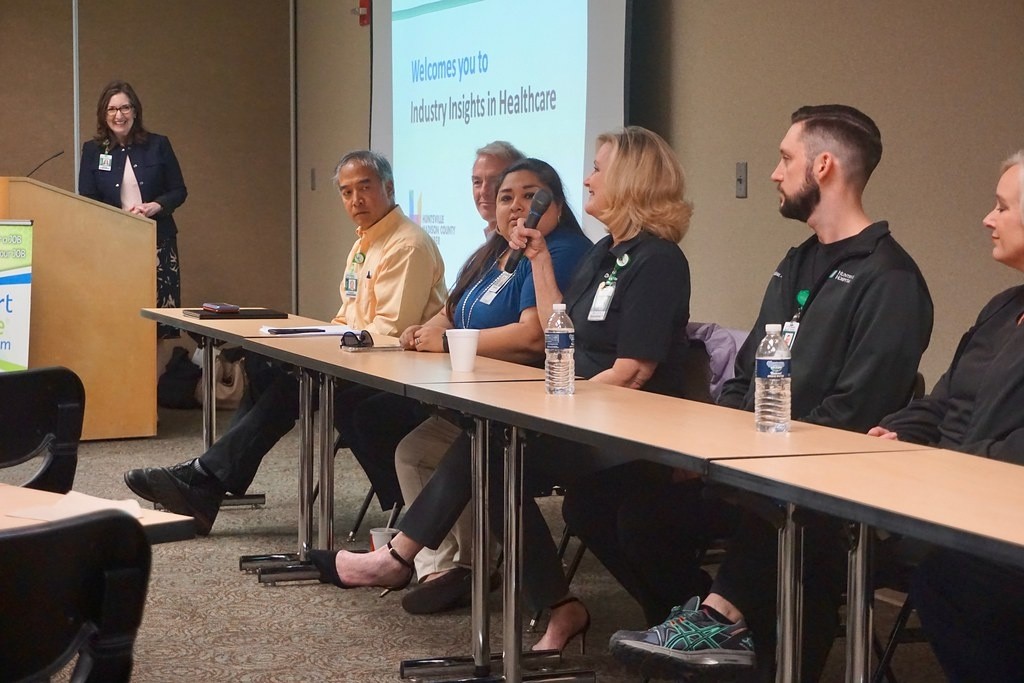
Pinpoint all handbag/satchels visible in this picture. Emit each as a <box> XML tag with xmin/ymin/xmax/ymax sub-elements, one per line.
<box><xmin>195</xmin><ymin>339</ymin><xmax>246</xmax><ymax>412</ymax></box>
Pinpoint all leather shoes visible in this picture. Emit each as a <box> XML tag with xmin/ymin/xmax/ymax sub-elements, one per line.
<box><xmin>123</xmin><ymin>461</ymin><xmax>225</xmax><ymax>536</ymax></box>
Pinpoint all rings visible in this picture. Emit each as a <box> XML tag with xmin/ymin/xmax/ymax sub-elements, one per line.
<box><xmin>415</xmin><ymin>338</ymin><xmax>420</xmax><ymax>345</ymax></box>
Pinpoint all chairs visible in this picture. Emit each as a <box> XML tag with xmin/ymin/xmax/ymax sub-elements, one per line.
<box><xmin>0</xmin><ymin>367</ymin><xmax>85</xmax><ymax>495</ymax></box>
<box><xmin>0</xmin><ymin>509</ymin><xmax>152</xmax><ymax>683</ymax></box>
<box><xmin>526</xmin><ymin>322</ymin><xmax>749</xmax><ymax>634</ymax></box>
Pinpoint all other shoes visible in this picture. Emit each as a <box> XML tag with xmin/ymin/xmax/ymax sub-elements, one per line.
<box><xmin>401</xmin><ymin>567</ymin><xmax>501</xmax><ymax>615</ymax></box>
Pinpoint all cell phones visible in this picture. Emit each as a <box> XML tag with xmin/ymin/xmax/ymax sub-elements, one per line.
<box><xmin>202</xmin><ymin>302</ymin><xmax>240</xmax><ymax>313</ymax></box>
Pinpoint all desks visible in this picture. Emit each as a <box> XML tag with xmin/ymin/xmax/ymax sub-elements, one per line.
<box><xmin>140</xmin><ymin>307</ymin><xmax>1024</xmax><ymax>683</ymax></box>
<box><xmin>0</xmin><ymin>481</ymin><xmax>196</xmax><ymax>545</ymax></box>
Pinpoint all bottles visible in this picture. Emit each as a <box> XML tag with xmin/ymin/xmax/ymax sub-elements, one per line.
<box><xmin>754</xmin><ymin>323</ymin><xmax>791</xmax><ymax>433</ymax></box>
<box><xmin>544</xmin><ymin>304</ymin><xmax>576</xmax><ymax>396</ymax></box>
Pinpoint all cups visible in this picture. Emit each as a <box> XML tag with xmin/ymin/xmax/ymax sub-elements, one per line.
<box><xmin>370</xmin><ymin>528</ymin><xmax>399</xmax><ymax>553</ymax></box>
<box><xmin>444</xmin><ymin>329</ymin><xmax>480</xmax><ymax>372</ymax></box>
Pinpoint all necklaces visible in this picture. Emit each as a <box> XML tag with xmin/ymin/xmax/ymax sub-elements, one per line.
<box><xmin>462</xmin><ymin>244</ymin><xmax>510</xmax><ymax>329</ymax></box>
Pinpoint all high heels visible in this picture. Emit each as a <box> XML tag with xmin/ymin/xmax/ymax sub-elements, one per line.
<box><xmin>521</xmin><ymin>598</ymin><xmax>591</xmax><ymax>655</ymax></box>
<box><xmin>304</xmin><ymin>542</ymin><xmax>416</xmax><ymax>591</ymax></box>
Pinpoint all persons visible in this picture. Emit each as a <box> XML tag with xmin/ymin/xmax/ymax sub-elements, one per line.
<box><xmin>123</xmin><ymin>149</ymin><xmax>451</xmax><ymax>534</ymax></box>
<box><xmin>337</xmin><ymin>141</ymin><xmax>526</xmax><ymax>510</ymax></box>
<box><xmin>78</xmin><ymin>79</ymin><xmax>188</xmax><ymax>422</ymax></box>
<box><xmin>396</xmin><ymin>156</ymin><xmax>591</xmax><ymax>616</ymax></box>
<box><xmin>561</xmin><ymin>100</ymin><xmax>1022</xmax><ymax>682</ymax></box>
<box><xmin>305</xmin><ymin>125</ymin><xmax>694</xmax><ymax>657</ymax></box>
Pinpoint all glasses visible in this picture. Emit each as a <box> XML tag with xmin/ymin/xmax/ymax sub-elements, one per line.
<box><xmin>341</xmin><ymin>330</ymin><xmax>374</xmax><ymax>347</ymax></box>
<box><xmin>105</xmin><ymin>104</ymin><xmax>134</xmax><ymax>115</ymax></box>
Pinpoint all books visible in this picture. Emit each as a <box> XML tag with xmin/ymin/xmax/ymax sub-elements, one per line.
<box><xmin>183</xmin><ymin>308</ymin><xmax>289</xmax><ymax>320</ymax></box>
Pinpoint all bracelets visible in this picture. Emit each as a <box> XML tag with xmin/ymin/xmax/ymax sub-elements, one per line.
<box><xmin>442</xmin><ymin>332</ymin><xmax>449</xmax><ymax>353</ymax></box>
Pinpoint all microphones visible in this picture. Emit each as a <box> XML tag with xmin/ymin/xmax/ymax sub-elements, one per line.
<box><xmin>26</xmin><ymin>151</ymin><xmax>65</xmax><ymax>178</ymax></box>
<box><xmin>505</xmin><ymin>189</ymin><xmax>554</xmax><ymax>273</ymax></box>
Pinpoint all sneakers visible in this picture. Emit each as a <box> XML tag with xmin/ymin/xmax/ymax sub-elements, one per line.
<box><xmin>605</xmin><ymin>596</ymin><xmax>757</xmax><ymax>677</ymax></box>
